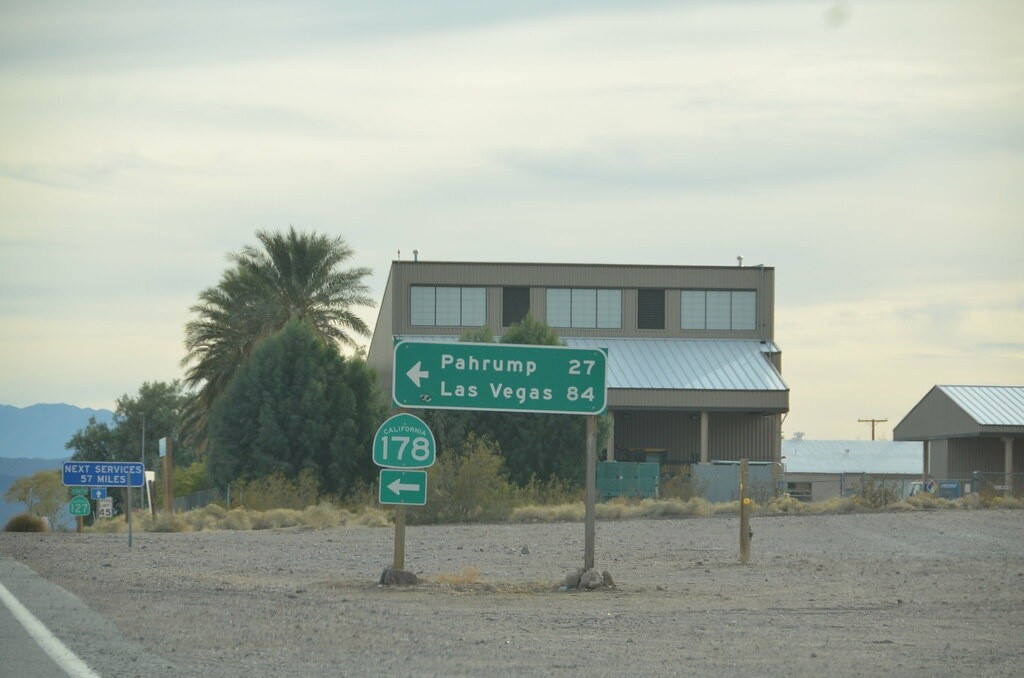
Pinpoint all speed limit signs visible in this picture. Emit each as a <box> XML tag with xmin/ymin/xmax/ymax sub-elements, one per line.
<box><xmin>98</xmin><ymin>497</ymin><xmax>114</xmax><ymax>519</ymax></box>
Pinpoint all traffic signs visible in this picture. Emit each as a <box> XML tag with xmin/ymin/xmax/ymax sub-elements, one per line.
<box><xmin>390</xmin><ymin>340</ymin><xmax>609</xmax><ymax>416</ymax></box>
